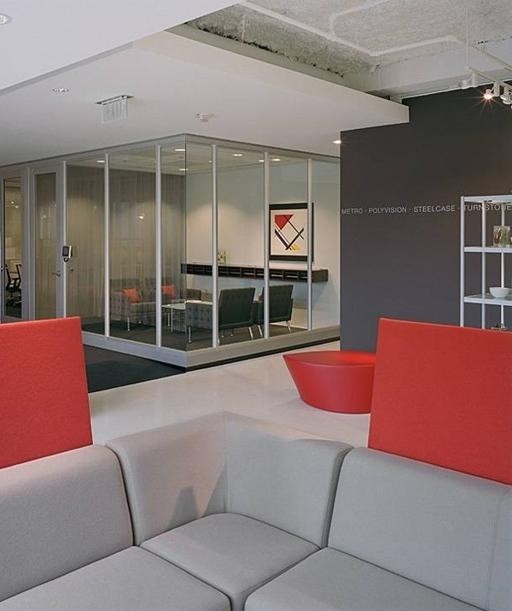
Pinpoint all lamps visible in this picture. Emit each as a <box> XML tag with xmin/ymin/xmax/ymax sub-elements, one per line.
<box><xmin>458</xmin><ymin>68</ymin><xmax>512</xmax><ymax>106</ymax></box>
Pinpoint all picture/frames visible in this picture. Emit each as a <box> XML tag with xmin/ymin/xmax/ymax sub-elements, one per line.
<box><xmin>269</xmin><ymin>202</ymin><xmax>314</xmax><ymax>260</ymax></box>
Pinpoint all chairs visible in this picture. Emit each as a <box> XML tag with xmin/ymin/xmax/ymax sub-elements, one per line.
<box><xmin>282</xmin><ymin>350</ymin><xmax>376</xmax><ymax>414</ymax></box>
<box><xmin>104</xmin><ymin>276</ymin><xmax>295</xmax><ymax>344</ymax></box>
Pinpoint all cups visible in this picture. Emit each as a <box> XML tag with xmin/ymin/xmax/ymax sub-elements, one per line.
<box><xmin>492</xmin><ymin>223</ymin><xmax>511</xmax><ymax>247</ymax></box>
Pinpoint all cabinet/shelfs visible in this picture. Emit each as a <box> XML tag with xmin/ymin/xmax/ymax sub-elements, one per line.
<box><xmin>459</xmin><ymin>195</ymin><xmax>512</xmax><ymax>330</ymax></box>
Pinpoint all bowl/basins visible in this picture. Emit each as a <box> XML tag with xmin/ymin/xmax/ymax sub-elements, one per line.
<box><xmin>489</xmin><ymin>286</ymin><xmax>511</xmax><ymax>298</ymax></box>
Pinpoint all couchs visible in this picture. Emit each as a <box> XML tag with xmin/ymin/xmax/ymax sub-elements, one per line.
<box><xmin>0</xmin><ymin>411</ymin><xmax>512</xmax><ymax>611</ymax></box>
